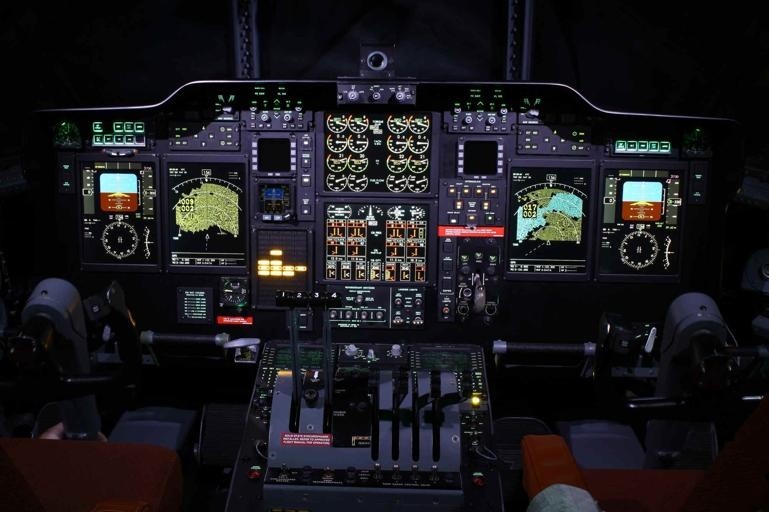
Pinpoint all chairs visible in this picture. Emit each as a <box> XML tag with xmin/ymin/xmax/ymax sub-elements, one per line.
<box><xmin>0</xmin><ymin>434</ymin><xmax>185</xmax><ymax>512</ymax></box>
<box><xmin>520</xmin><ymin>434</ymin><xmax>767</xmax><ymax>511</ymax></box>
